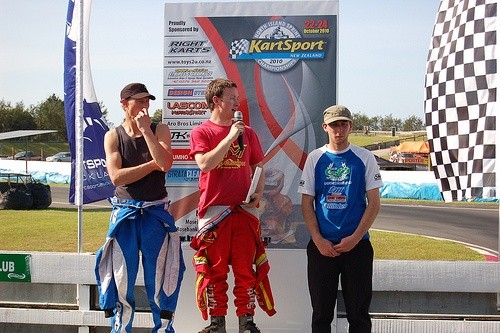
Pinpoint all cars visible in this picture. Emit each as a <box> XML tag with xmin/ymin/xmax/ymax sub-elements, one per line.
<box><xmin>14</xmin><ymin>150</ymin><xmax>34</xmax><ymax>160</ymax></box>
<box><xmin>389</xmin><ymin>152</ymin><xmax>423</xmax><ymax>164</ymax></box>
<box><xmin>45</xmin><ymin>151</ymin><xmax>72</xmax><ymax>163</ymax></box>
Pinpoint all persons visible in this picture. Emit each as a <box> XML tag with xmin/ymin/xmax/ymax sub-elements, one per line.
<box><xmin>297</xmin><ymin>105</ymin><xmax>384</xmax><ymax>333</ymax></box>
<box><xmin>103</xmin><ymin>83</ymin><xmax>173</xmax><ymax>333</ymax></box>
<box><xmin>190</xmin><ymin>78</ymin><xmax>266</xmax><ymax>333</ymax></box>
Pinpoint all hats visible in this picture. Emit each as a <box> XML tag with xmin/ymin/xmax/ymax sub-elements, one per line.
<box><xmin>323</xmin><ymin>104</ymin><xmax>354</xmax><ymax>125</ymax></box>
<box><xmin>120</xmin><ymin>83</ymin><xmax>156</xmax><ymax>103</ymax></box>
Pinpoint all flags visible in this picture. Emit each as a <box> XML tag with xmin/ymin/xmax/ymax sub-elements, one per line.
<box><xmin>423</xmin><ymin>0</ymin><xmax>500</xmax><ymax>203</ymax></box>
<box><xmin>65</xmin><ymin>0</ymin><xmax>116</xmax><ymax>203</ymax></box>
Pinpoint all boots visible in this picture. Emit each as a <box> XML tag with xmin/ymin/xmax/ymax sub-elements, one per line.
<box><xmin>198</xmin><ymin>316</ymin><xmax>227</xmax><ymax>333</ymax></box>
<box><xmin>239</xmin><ymin>314</ymin><xmax>262</xmax><ymax>333</ymax></box>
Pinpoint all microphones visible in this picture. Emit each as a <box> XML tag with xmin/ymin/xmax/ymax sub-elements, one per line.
<box><xmin>234</xmin><ymin>111</ymin><xmax>243</xmax><ymax>150</ymax></box>
